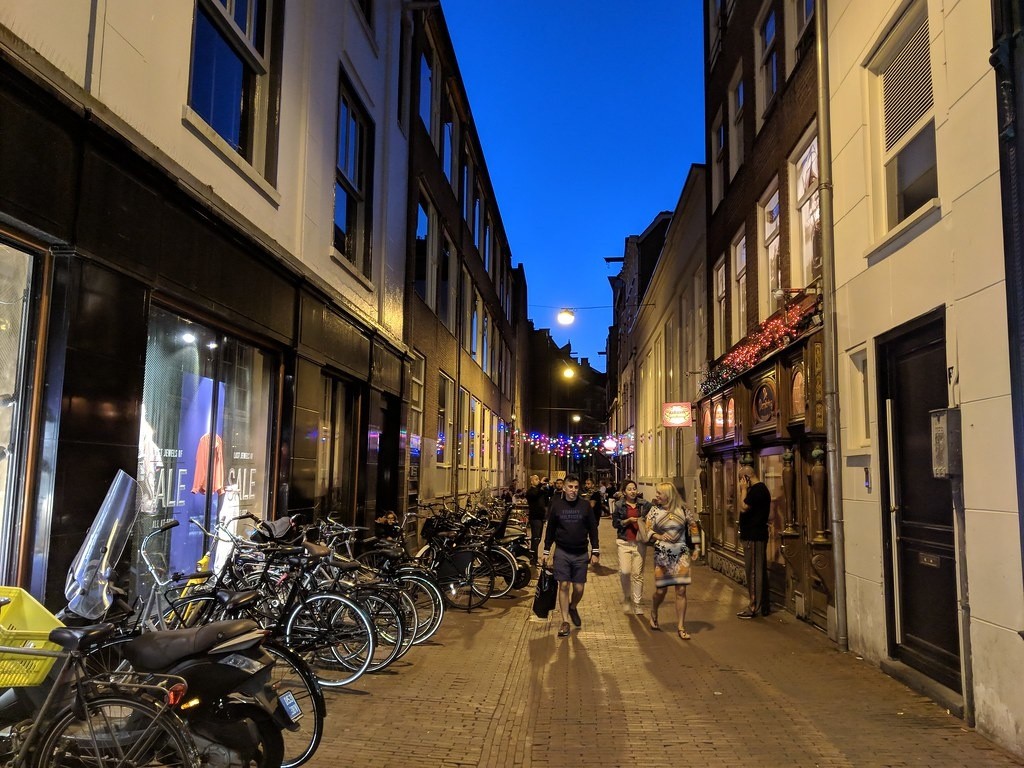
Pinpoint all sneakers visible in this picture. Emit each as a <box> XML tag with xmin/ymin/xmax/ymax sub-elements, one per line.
<box><xmin>568</xmin><ymin>603</ymin><xmax>581</xmax><ymax>626</ymax></box>
<box><xmin>634</xmin><ymin>602</ymin><xmax>644</xmax><ymax>615</ymax></box>
<box><xmin>623</xmin><ymin>601</ymin><xmax>632</xmax><ymax>614</ymax></box>
<box><xmin>558</xmin><ymin>622</ymin><xmax>570</xmax><ymax>637</ymax></box>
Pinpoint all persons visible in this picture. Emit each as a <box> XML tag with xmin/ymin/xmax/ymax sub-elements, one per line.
<box><xmin>735</xmin><ymin>466</ymin><xmax>771</xmax><ymax>619</ymax></box>
<box><xmin>525</xmin><ymin>475</ymin><xmax>549</xmax><ymax>566</ymax></box>
<box><xmin>645</xmin><ymin>482</ymin><xmax>701</xmax><ymax>639</ymax></box>
<box><xmin>584</xmin><ymin>478</ymin><xmax>602</xmax><ymax>564</ymax></box>
<box><xmin>612</xmin><ymin>480</ymin><xmax>650</xmax><ymax>615</ymax></box>
<box><xmin>501</xmin><ymin>479</ymin><xmax>621</xmax><ymax>517</ymax></box>
<box><xmin>541</xmin><ymin>473</ymin><xmax>600</xmax><ymax>636</ymax></box>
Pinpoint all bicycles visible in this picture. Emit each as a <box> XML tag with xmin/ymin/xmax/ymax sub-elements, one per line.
<box><xmin>0</xmin><ymin>598</ymin><xmax>203</xmax><ymax>768</ymax></box>
<box><xmin>109</xmin><ymin>491</ymin><xmax>535</xmax><ymax>768</ymax></box>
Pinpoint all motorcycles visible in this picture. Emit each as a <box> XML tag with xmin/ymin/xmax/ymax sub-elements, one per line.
<box><xmin>0</xmin><ymin>468</ymin><xmax>304</xmax><ymax>768</ymax></box>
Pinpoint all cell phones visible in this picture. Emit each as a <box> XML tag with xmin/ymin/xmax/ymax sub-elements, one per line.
<box><xmin>745</xmin><ymin>475</ymin><xmax>750</xmax><ymax>482</ymax></box>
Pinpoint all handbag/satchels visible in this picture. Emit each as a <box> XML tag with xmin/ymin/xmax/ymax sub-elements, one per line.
<box><xmin>533</xmin><ymin>566</ymin><xmax>558</xmax><ymax>619</ymax></box>
<box><xmin>683</xmin><ymin>508</ymin><xmax>702</xmax><ymax>550</ymax></box>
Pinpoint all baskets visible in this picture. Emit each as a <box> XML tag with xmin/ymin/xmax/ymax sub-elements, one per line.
<box><xmin>0</xmin><ymin>586</ymin><xmax>68</xmax><ymax>688</ymax></box>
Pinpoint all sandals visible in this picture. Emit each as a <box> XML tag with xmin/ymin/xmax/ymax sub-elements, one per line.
<box><xmin>677</xmin><ymin>627</ymin><xmax>691</xmax><ymax>640</ymax></box>
<box><xmin>650</xmin><ymin>610</ymin><xmax>658</xmax><ymax>629</ymax></box>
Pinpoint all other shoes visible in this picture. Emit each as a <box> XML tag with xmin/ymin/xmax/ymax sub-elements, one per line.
<box><xmin>736</xmin><ymin>606</ymin><xmax>758</xmax><ymax>619</ymax></box>
<box><xmin>530</xmin><ymin>560</ymin><xmax>541</xmax><ymax>567</ymax></box>
